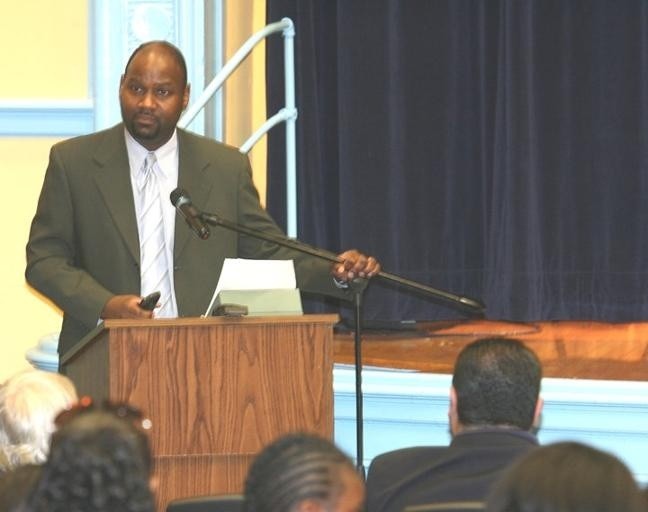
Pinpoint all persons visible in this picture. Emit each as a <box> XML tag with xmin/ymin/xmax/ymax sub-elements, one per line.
<box><xmin>481</xmin><ymin>439</ymin><xmax>647</xmax><ymax>510</ymax></box>
<box><xmin>245</xmin><ymin>431</ymin><xmax>366</xmax><ymax>511</ymax></box>
<box><xmin>0</xmin><ymin>369</ymin><xmax>78</xmax><ymax>511</ymax></box>
<box><xmin>363</xmin><ymin>335</ymin><xmax>548</xmax><ymax>510</ymax></box>
<box><xmin>23</xmin><ymin>39</ymin><xmax>380</xmax><ymax>415</ymax></box>
<box><xmin>19</xmin><ymin>397</ymin><xmax>160</xmax><ymax>512</ymax></box>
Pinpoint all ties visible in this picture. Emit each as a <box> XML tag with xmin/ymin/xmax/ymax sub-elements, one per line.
<box><xmin>137</xmin><ymin>151</ymin><xmax>174</xmax><ymax>320</ymax></box>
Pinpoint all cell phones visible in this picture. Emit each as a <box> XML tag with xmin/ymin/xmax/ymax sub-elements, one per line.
<box><xmin>139</xmin><ymin>292</ymin><xmax>161</xmax><ymax>310</ymax></box>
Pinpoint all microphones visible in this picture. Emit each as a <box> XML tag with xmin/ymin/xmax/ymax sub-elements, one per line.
<box><xmin>169</xmin><ymin>187</ymin><xmax>211</xmax><ymax>241</ymax></box>
